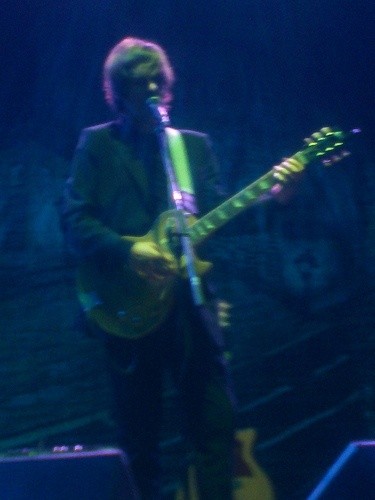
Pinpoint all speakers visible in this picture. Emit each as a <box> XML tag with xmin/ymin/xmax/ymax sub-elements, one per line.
<box><xmin>302</xmin><ymin>441</ymin><xmax>375</xmax><ymax>499</ymax></box>
<box><xmin>1</xmin><ymin>445</ymin><xmax>131</xmax><ymax>500</ymax></box>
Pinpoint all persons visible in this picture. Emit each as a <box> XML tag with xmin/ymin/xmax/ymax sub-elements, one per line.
<box><xmin>59</xmin><ymin>35</ymin><xmax>306</xmax><ymax>500</ymax></box>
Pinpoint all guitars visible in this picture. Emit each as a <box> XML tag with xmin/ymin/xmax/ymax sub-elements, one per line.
<box><xmin>175</xmin><ymin>300</ymin><xmax>271</xmax><ymax>500</ymax></box>
<box><xmin>75</xmin><ymin>126</ymin><xmax>362</xmax><ymax>336</ymax></box>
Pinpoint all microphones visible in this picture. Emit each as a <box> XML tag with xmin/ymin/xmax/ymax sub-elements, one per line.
<box><xmin>147</xmin><ymin>96</ymin><xmax>170</xmax><ymax>127</ymax></box>
